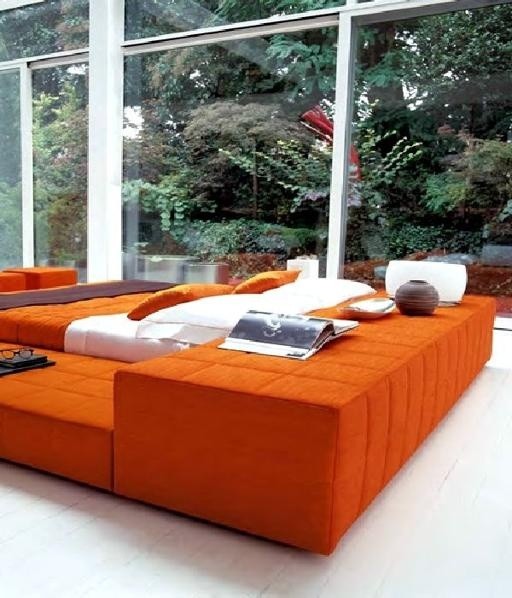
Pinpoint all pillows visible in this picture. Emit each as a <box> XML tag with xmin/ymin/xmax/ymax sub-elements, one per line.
<box><xmin>135</xmin><ymin>295</ymin><xmax>294</xmax><ymax>345</ymax></box>
<box><xmin>261</xmin><ymin>279</ymin><xmax>377</xmax><ymax>316</ymax></box>
<box><xmin>234</xmin><ymin>271</ymin><xmax>301</xmax><ymax>294</ymax></box>
<box><xmin>127</xmin><ymin>284</ymin><xmax>233</xmax><ymax>321</ymax></box>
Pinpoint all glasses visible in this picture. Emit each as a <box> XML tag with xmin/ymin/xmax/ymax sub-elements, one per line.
<box><xmin>0</xmin><ymin>349</ymin><xmax>35</xmax><ymax>361</ymax></box>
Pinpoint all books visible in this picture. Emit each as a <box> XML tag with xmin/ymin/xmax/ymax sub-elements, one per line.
<box><xmin>216</xmin><ymin>308</ymin><xmax>362</xmax><ymax>362</ymax></box>
<box><xmin>0</xmin><ymin>359</ymin><xmax>57</xmax><ymax>377</ymax></box>
<box><xmin>1</xmin><ymin>353</ymin><xmax>49</xmax><ymax>369</ymax></box>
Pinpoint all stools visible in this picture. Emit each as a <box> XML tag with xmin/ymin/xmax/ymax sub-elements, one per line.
<box><xmin>5</xmin><ymin>267</ymin><xmax>78</xmax><ymax>288</ymax></box>
<box><xmin>0</xmin><ymin>273</ymin><xmax>25</xmax><ymax>293</ymax></box>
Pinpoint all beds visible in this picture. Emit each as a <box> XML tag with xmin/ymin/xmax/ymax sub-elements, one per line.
<box><xmin>0</xmin><ymin>281</ymin><xmax>496</xmax><ymax>556</ymax></box>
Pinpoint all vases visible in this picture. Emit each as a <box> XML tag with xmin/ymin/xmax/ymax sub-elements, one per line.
<box><xmin>396</xmin><ymin>281</ymin><xmax>439</xmax><ymax>316</ymax></box>
